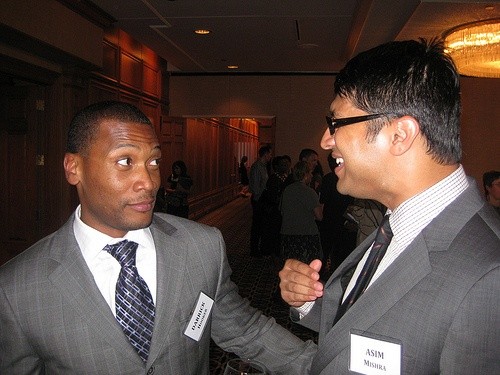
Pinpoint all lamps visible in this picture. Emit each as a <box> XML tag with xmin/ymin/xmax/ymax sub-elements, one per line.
<box><xmin>443</xmin><ymin>19</ymin><xmax>500</xmax><ymax>79</ymax></box>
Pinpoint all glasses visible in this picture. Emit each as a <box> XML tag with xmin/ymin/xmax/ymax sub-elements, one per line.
<box><xmin>326</xmin><ymin>114</ymin><xmax>388</xmax><ymax>135</ymax></box>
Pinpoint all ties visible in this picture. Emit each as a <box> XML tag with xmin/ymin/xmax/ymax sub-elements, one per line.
<box><xmin>103</xmin><ymin>240</ymin><xmax>155</xmax><ymax>366</ymax></box>
<box><xmin>332</xmin><ymin>214</ymin><xmax>393</xmax><ymax>327</ymax></box>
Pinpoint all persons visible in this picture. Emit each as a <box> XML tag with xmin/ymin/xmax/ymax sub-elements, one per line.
<box><xmin>279</xmin><ymin>160</ymin><xmax>325</xmax><ymax>282</ymax></box>
<box><xmin>317</xmin><ymin>152</ymin><xmax>358</xmax><ymax>283</ymax></box>
<box><xmin>271</xmin><ymin>148</ymin><xmax>322</xmax><ymax>266</ymax></box>
<box><xmin>249</xmin><ymin>146</ymin><xmax>273</xmax><ymax>259</ymax></box>
<box><xmin>0</xmin><ymin>101</ymin><xmax>318</xmax><ymax>375</ymax></box>
<box><xmin>483</xmin><ymin>171</ymin><xmax>500</xmax><ymax>215</ymax></box>
<box><xmin>278</xmin><ymin>40</ymin><xmax>500</xmax><ymax>375</ymax></box>
<box><xmin>165</xmin><ymin>160</ymin><xmax>193</xmax><ymax>219</ymax></box>
<box><xmin>239</xmin><ymin>156</ymin><xmax>249</xmax><ymax>199</ymax></box>
